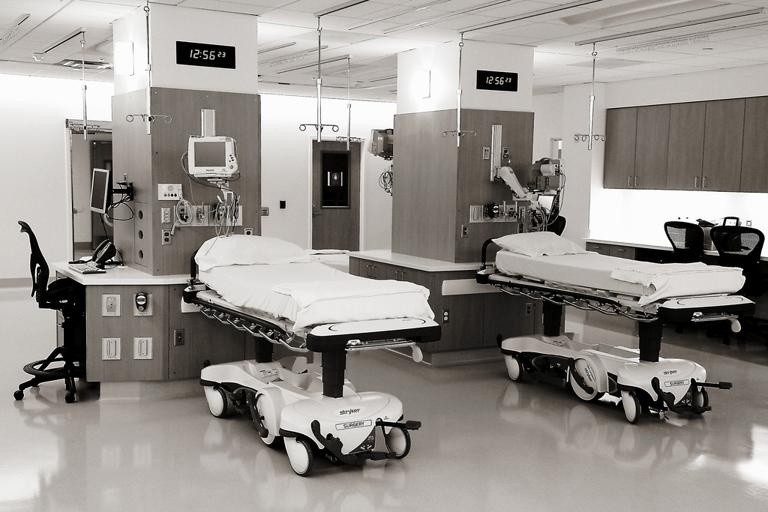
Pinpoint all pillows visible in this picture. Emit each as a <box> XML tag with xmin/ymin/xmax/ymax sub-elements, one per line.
<box><xmin>195</xmin><ymin>232</ymin><xmax>310</xmax><ymax>271</ymax></box>
<box><xmin>492</xmin><ymin>230</ymin><xmax>585</xmax><ymax>257</ymax></box>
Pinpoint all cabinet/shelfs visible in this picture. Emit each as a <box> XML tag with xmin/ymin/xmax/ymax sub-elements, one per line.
<box><xmin>585</xmin><ymin>242</ymin><xmax>635</xmax><ymax>260</ymax></box>
<box><xmin>740</xmin><ymin>97</ymin><xmax>768</xmax><ymax>192</ymax></box>
<box><xmin>603</xmin><ymin>104</ymin><xmax>665</xmax><ymax>190</ymax></box>
<box><xmin>666</xmin><ymin>98</ymin><xmax>747</xmax><ymax>192</ymax></box>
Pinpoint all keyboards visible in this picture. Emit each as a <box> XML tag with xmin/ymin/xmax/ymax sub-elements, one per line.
<box><xmin>68</xmin><ymin>263</ymin><xmax>108</xmax><ymax>276</ymax></box>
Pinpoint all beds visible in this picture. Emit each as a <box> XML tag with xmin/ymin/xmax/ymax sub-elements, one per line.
<box><xmin>478</xmin><ymin>231</ymin><xmax>752</xmax><ymax>421</ymax></box>
<box><xmin>179</xmin><ymin>234</ymin><xmax>442</xmax><ymax>477</ymax></box>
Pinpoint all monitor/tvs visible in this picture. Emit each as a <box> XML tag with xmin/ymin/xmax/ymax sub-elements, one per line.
<box><xmin>88</xmin><ymin>166</ymin><xmax>112</xmax><ymax>216</ymax></box>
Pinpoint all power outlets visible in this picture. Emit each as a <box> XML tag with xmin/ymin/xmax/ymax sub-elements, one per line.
<box><xmin>209</xmin><ymin>205</ymin><xmax>227</xmax><ymax>227</ymax></box>
<box><xmin>174</xmin><ymin>205</ymin><xmax>195</xmax><ymax>227</ymax></box>
<box><xmin>505</xmin><ymin>205</ymin><xmax>516</xmax><ymax>222</ymax></box>
<box><xmin>483</xmin><ymin>204</ymin><xmax>505</xmax><ymax>222</ymax></box>
<box><xmin>519</xmin><ymin>206</ymin><xmax>526</xmax><ymax>220</ymax></box>
<box><xmin>226</xmin><ymin>205</ymin><xmax>243</xmax><ymax>226</ymax></box>
<box><xmin>195</xmin><ymin>205</ymin><xmax>209</xmax><ymax>227</ymax></box>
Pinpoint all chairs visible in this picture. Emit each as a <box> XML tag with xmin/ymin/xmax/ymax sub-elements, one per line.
<box><xmin>711</xmin><ymin>226</ymin><xmax>764</xmax><ymax>295</ymax></box>
<box><xmin>14</xmin><ymin>220</ymin><xmax>85</xmax><ymax>402</ymax></box>
<box><xmin>664</xmin><ymin>222</ymin><xmax>704</xmax><ymax>261</ymax></box>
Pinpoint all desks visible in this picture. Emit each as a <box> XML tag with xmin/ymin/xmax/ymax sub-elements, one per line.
<box><xmin>637</xmin><ymin>245</ymin><xmax>768</xmax><ymax>265</ymax></box>
<box><xmin>56</xmin><ymin>258</ymin><xmax>167</xmax><ymax>382</ymax></box>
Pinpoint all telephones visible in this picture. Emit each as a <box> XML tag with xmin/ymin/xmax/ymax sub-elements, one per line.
<box><xmin>91</xmin><ymin>238</ymin><xmax>116</xmax><ymax>263</ymax></box>
